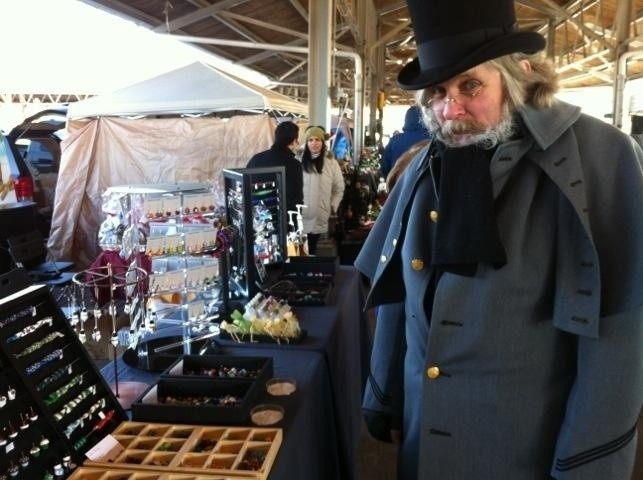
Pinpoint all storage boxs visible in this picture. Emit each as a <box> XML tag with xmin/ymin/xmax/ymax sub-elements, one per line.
<box><xmin>285</xmin><ymin>256</ymin><xmax>340</xmax><ymax>279</ymax></box>
<box><xmin>131</xmin><ymin>378</ymin><xmax>261</xmax><ymax>427</ymax></box>
<box><xmin>160</xmin><ymin>354</ymin><xmax>273</xmax><ymax>405</ymax></box>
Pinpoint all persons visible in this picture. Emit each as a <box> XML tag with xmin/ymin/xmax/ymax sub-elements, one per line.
<box><xmin>298</xmin><ymin>123</ymin><xmax>346</xmax><ymax>254</ymax></box>
<box><xmin>380</xmin><ymin>105</ymin><xmax>427</xmax><ymax>190</ymax></box>
<box><xmin>352</xmin><ymin>0</ymin><xmax>642</xmax><ymax>478</ymax></box>
<box><xmin>247</xmin><ymin>121</ymin><xmax>305</xmax><ymax>234</ymax></box>
<box><xmin>385</xmin><ymin>137</ymin><xmax>431</xmax><ymax>190</ymax></box>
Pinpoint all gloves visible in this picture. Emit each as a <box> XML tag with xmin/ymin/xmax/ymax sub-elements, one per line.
<box><xmin>361</xmin><ymin>407</ymin><xmax>398</xmax><ymax>447</ymax></box>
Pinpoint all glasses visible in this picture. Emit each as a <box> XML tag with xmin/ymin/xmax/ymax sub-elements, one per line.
<box><xmin>425</xmin><ymin>69</ymin><xmax>504</xmax><ymax>111</ymax></box>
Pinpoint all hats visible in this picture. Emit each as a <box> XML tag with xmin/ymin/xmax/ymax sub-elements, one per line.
<box><xmin>403</xmin><ymin>105</ymin><xmax>428</xmax><ymax>132</ymax></box>
<box><xmin>392</xmin><ymin>0</ymin><xmax>548</xmax><ymax>91</ymax></box>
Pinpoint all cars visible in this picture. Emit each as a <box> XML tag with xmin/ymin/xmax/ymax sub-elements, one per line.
<box><xmin>0</xmin><ymin>107</ymin><xmax>66</xmax><ymax>220</ymax></box>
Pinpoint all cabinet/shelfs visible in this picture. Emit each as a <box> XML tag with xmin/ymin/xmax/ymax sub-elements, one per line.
<box><xmin>223</xmin><ymin>167</ymin><xmax>287</xmax><ymax>300</ymax></box>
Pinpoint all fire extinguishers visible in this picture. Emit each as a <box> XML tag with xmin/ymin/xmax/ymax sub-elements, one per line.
<box><xmin>12</xmin><ymin>166</ymin><xmax>34</xmax><ymax>203</ymax></box>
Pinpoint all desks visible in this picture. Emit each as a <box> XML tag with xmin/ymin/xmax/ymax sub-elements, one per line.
<box><xmin>157</xmin><ymin>264</ymin><xmax>373</xmax><ymax>459</ymax></box>
<box><xmin>99</xmin><ymin>346</ymin><xmax>354</xmax><ymax>480</ymax></box>
